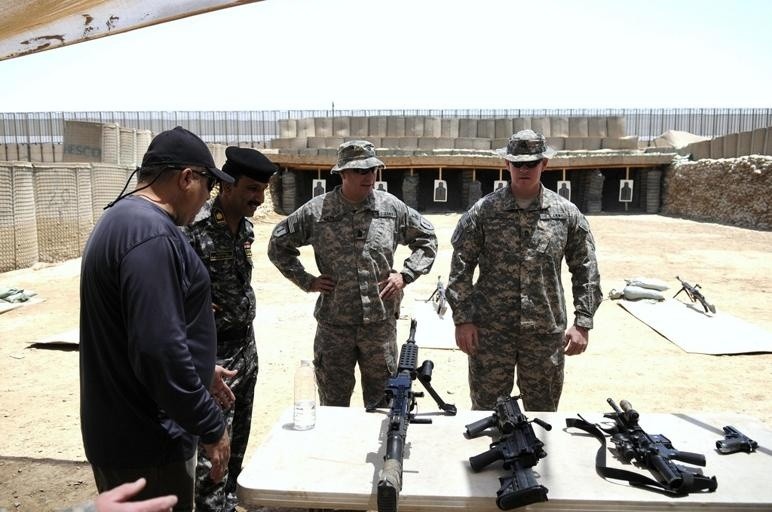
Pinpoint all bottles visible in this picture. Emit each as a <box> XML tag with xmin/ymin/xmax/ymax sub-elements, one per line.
<box><xmin>293</xmin><ymin>359</ymin><xmax>317</xmax><ymax>430</ymax></box>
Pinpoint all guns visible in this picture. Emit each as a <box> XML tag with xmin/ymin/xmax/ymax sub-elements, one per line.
<box><xmin>464</xmin><ymin>386</ymin><xmax>555</xmax><ymax>512</ymax></box>
<box><xmin>603</xmin><ymin>394</ymin><xmax>718</xmax><ymax>495</ymax></box>
<box><xmin>718</xmin><ymin>423</ymin><xmax>759</xmax><ymax>456</ymax></box>
<box><xmin>366</xmin><ymin>317</ymin><xmax>458</xmax><ymax>512</ymax></box>
<box><xmin>426</xmin><ymin>274</ymin><xmax>450</xmax><ymax>317</ymax></box>
<box><xmin>671</xmin><ymin>276</ymin><xmax>719</xmax><ymax>316</ymax></box>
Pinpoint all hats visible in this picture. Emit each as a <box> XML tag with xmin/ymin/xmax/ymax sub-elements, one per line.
<box><xmin>141</xmin><ymin>126</ymin><xmax>235</xmax><ymax>183</ymax></box>
<box><xmin>226</xmin><ymin>146</ymin><xmax>279</xmax><ymax>183</ymax></box>
<box><xmin>330</xmin><ymin>140</ymin><xmax>384</xmax><ymax>174</ymax></box>
<box><xmin>498</xmin><ymin>129</ymin><xmax>555</xmax><ymax>162</ymax></box>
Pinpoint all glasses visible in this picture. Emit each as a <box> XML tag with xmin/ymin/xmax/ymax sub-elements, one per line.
<box><xmin>512</xmin><ymin>159</ymin><xmax>542</xmax><ymax>168</ymax></box>
<box><xmin>168</xmin><ymin>166</ymin><xmax>218</xmax><ymax>190</ymax></box>
<box><xmin>346</xmin><ymin>166</ymin><xmax>377</xmax><ymax>174</ymax></box>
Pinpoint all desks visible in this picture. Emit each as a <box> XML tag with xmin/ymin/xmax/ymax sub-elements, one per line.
<box><xmin>237</xmin><ymin>403</ymin><xmax>770</xmax><ymax>511</ymax></box>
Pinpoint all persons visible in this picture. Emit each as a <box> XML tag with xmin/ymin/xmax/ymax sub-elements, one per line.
<box><xmin>76</xmin><ymin>124</ymin><xmax>239</xmax><ymax>512</ymax></box>
<box><xmin>182</xmin><ymin>145</ymin><xmax>277</xmax><ymax>512</ymax></box>
<box><xmin>57</xmin><ymin>475</ymin><xmax>180</xmax><ymax>512</ymax></box>
<box><xmin>443</xmin><ymin>129</ymin><xmax>603</xmax><ymax>413</ymax></box>
<box><xmin>265</xmin><ymin>139</ymin><xmax>440</xmax><ymax>409</ymax></box>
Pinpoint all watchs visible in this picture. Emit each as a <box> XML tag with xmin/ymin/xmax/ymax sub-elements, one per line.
<box><xmin>400</xmin><ymin>272</ymin><xmax>413</xmax><ymax>284</ymax></box>
